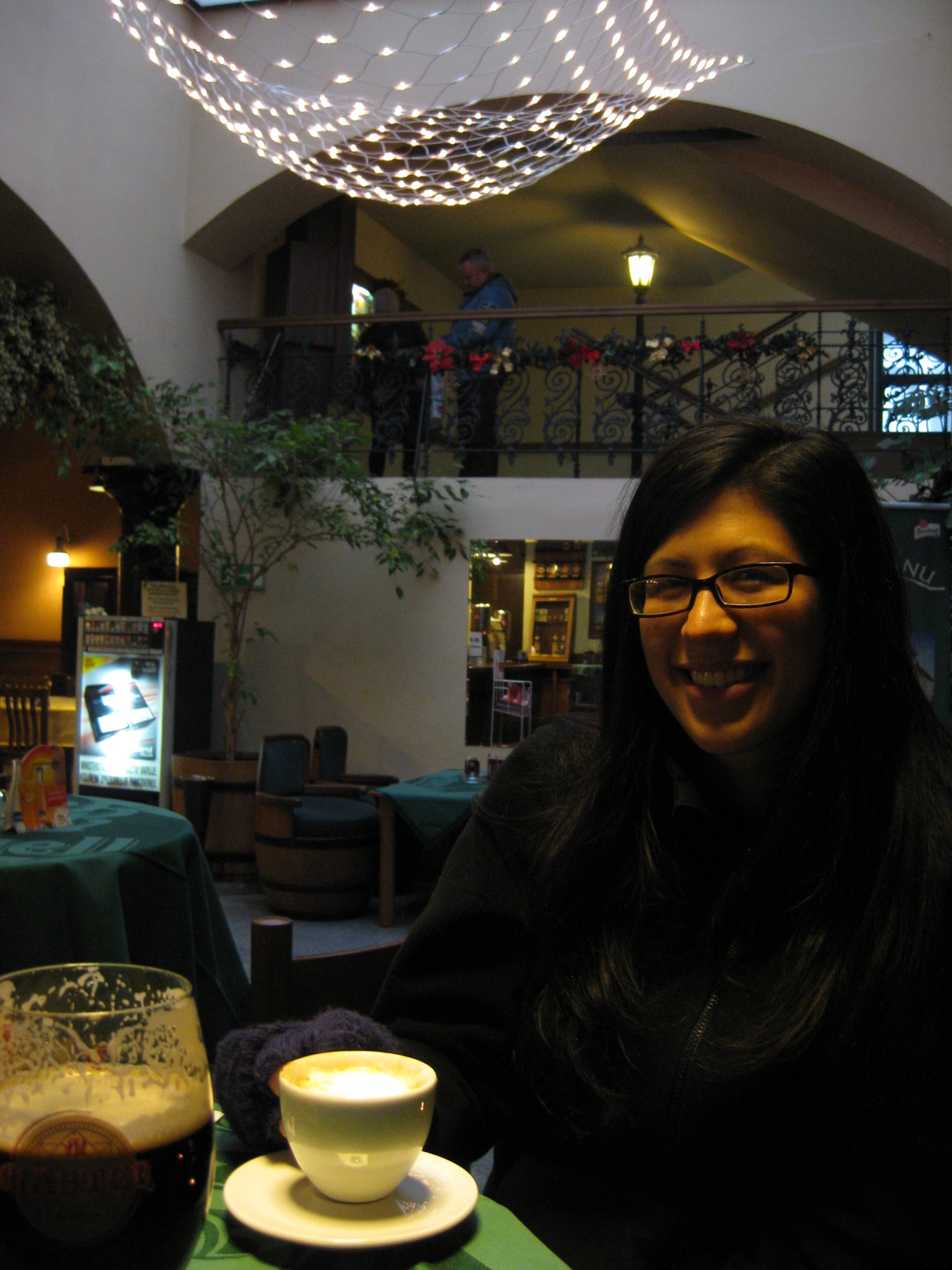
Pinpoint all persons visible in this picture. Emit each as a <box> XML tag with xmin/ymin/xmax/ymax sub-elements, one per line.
<box><xmin>435</xmin><ymin>246</ymin><xmax>519</xmax><ymax>477</ymax></box>
<box><xmin>211</xmin><ymin>418</ymin><xmax>952</xmax><ymax>1270</ymax></box>
<box><xmin>350</xmin><ymin>279</ymin><xmax>433</xmax><ymax>480</ymax></box>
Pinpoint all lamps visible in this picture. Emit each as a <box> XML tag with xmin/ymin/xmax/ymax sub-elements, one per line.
<box><xmin>45</xmin><ymin>528</ymin><xmax>72</xmax><ymax>567</ymax></box>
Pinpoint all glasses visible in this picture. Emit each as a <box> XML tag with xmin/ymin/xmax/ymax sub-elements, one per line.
<box><xmin>619</xmin><ymin>560</ymin><xmax>815</xmax><ymax>619</ymax></box>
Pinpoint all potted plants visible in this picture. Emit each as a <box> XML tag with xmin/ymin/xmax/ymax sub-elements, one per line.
<box><xmin>97</xmin><ymin>376</ymin><xmax>472</xmax><ymax>880</ymax></box>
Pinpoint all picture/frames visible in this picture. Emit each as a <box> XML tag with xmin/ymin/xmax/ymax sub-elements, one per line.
<box><xmin>587</xmin><ymin>560</ymin><xmax>613</xmax><ymax>639</ymax></box>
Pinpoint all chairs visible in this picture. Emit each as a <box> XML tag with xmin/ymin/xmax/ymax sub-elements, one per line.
<box><xmin>2</xmin><ymin>686</ymin><xmax>50</xmax><ymax>775</ymax></box>
<box><xmin>252</xmin><ymin>731</ymin><xmax>386</xmax><ymax>926</ymax></box>
<box><xmin>310</xmin><ymin>723</ymin><xmax>400</xmax><ymax>824</ymax></box>
<box><xmin>488</xmin><ymin>651</ymin><xmax>534</xmax><ymax>748</ymax></box>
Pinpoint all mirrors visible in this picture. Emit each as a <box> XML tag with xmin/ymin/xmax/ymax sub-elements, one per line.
<box><xmin>464</xmin><ymin>536</ymin><xmax>621</xmax><ymax>749</ymax></box>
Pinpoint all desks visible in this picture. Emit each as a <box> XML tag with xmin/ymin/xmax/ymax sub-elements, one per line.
<box><xmin>174</xmin><ymin>1093</ymin><xmax>573</xmax><ymax>1270</ymax></box>
<box><xmin>375</xmin><ymin>763</ymin><xmax>501</xmax><ymax>926</ymax></box>
<box><xmin>0</xmin><ymin>694</ymin><xmax>77</xmax><ymax>771</ymax></box>
<box><xmin>0</xmin><ymin>788</ymin><xmax>252</xmax><ymax>1029</ymax></box>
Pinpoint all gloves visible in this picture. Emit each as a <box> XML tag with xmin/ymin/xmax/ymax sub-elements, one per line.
<box><xmin>210</xmin><ymin>1007</ymin><xmax>400</xmax><ymax>1150</ymax></box>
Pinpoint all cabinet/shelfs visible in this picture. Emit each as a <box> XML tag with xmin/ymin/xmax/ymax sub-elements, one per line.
<box><xmin>566</xmin><ymin>661</ymin><xmax>602</xmax><ymax>715</ymax></box>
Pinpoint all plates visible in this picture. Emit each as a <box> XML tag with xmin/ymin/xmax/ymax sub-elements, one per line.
<box><xmin>219</xmin><ymin>1145</ymin><xmax>478</xmax><ymax>1252</ymax></box>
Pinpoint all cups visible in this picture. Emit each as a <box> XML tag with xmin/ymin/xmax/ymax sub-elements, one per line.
<box><xmin>2</xmin><ymin>961</ymin><xmax>211</xmax><ymax>1270</ymax></box>
<box><xmin>279</xmin><ymin>1047</ymin><xmax>437</xmax><ymax>1203</ymax></box>
